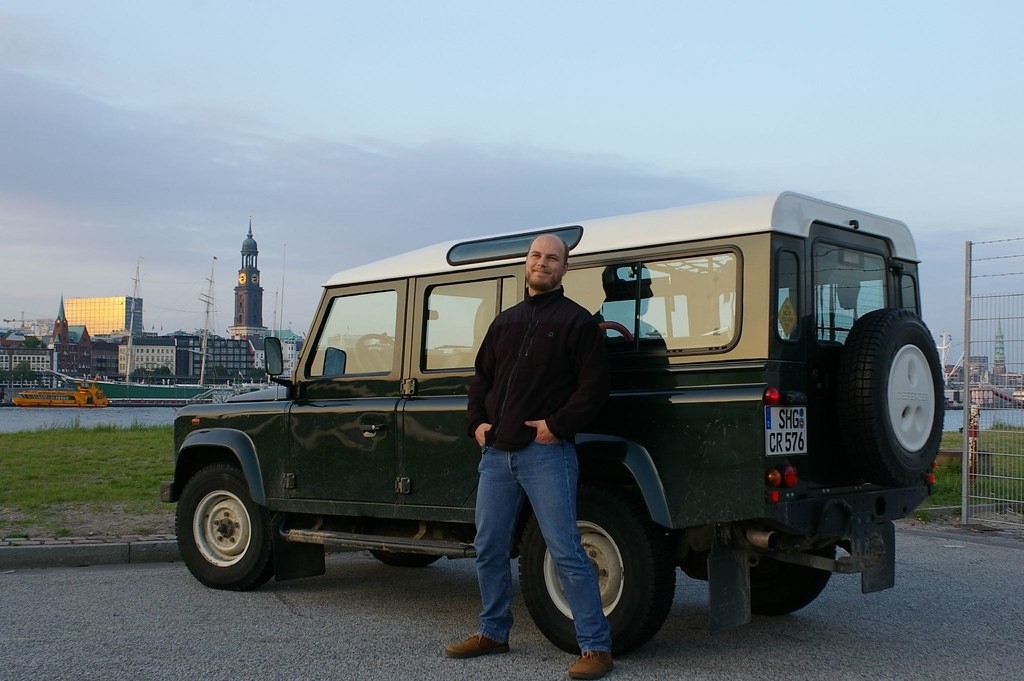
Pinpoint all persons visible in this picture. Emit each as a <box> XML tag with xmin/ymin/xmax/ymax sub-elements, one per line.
<box><xmin>590</xmin><ymin>257</ymin><xmax>677</xmax><ymax>449</ymax></box>
<box><xmin>444</xmin><ymin>234</ymin><xmax>615</xmax><ymax>681</ymax></box>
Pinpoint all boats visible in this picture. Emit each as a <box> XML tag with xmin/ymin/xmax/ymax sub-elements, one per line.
<box><xmin>10</xmin><ymin>378</ymin><xmax>110</xmax><ymax>408</ymax></box>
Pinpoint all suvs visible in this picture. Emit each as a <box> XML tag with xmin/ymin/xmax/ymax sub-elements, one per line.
<box><xmin>168</xmin><ymin>189</ymin><xmax>947</xmax><ymax>661</ymax></box>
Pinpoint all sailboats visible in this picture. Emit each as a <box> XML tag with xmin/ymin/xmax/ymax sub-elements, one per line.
<box><xmin>51</xmin><ymin>255</ymin><xmax>281</xmax><ymax>399</ymax></box>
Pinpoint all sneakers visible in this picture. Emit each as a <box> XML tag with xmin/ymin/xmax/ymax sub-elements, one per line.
<box><xmin>445</xmin><ymin>634</ymin><xmax>509</xmax><ymax>656</ymax></box>
<box><xmin>569</xmin><ymin>650</ymin><xmax>614</xmax><ymax>679</ymax></box>
